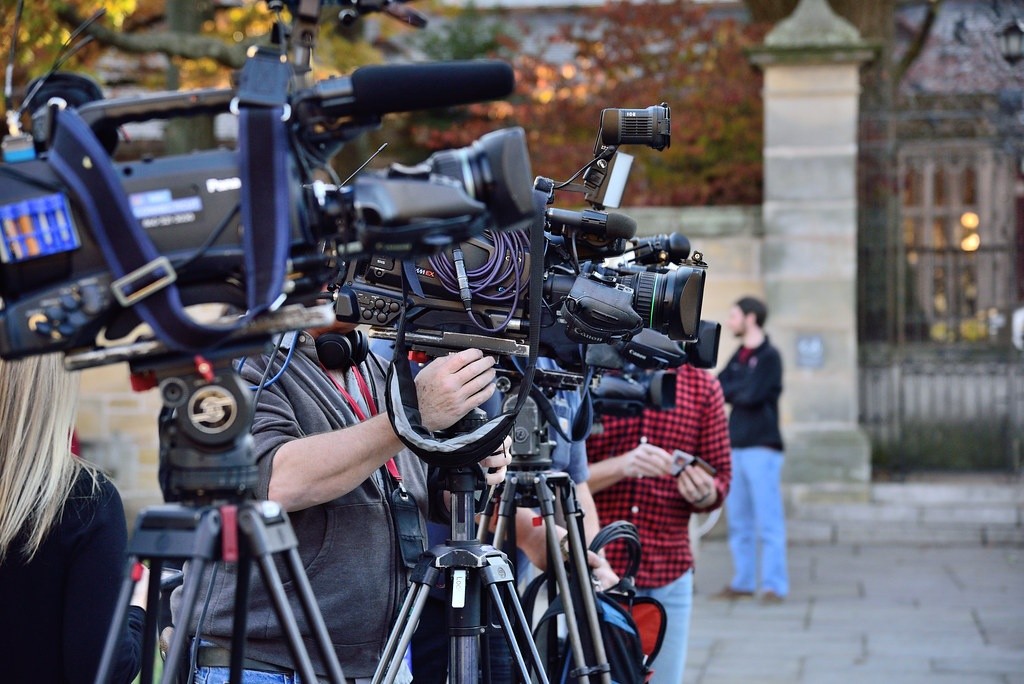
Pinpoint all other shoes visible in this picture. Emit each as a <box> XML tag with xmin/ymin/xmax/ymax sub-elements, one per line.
<box><xmin>714</xmin><ymin>588</ymin><xmax>753</xmax><ymax>599</ymax></box>
<box><xmin>760</xmin><ymin>591</ymin><xmax>784</xmax><ymax>603</ymax></box>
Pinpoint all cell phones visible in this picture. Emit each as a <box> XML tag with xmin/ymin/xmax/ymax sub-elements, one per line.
<box><xmin>674</xmin><ymin>457</ymin><xmax>717</xmax><ymax>479</ymax></box>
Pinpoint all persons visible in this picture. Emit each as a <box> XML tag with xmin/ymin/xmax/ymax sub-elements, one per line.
<box><xmin>716</xmin><ymin>297</ymin><xmax>788</xmax><ymax>605</ymax></box>
<box><xmin>155</xmin><ymin>243</ymin><xmax>733</xmax><ymax>683</ymax></box>
<box><xmin>0</xmin><ymin>351</ymin><xmax>152</xmax><ymax>684</ymax></box>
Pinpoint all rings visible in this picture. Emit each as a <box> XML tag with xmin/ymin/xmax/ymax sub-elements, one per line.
<box><xmin>703</xmin><ymin>489</ymin><xmax>711</xmax><ymax>497</ymax></box>
<box><xmin>696</xmin><ymin>498</ymin><xmax>704</xmax><ymax>503</ymax></box>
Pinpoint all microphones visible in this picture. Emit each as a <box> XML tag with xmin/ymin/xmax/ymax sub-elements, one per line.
<box><xmin>308</xmin><ymin>60</ymin><xmax>514</xmax><ymax>118</ymax></box>
<box><xmin>547</xmin><ymin>207</ymin><xmax>638</xmax><ymax>238</ymax></box>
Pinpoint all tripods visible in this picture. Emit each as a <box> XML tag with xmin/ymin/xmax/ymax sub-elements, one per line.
<box><xmin>96</xmin><ymin>335</ymin><xmax>347</xmax><ymax>684</ymax></box>
<box><xmin>370</xmin><ymin>369</ymin><xmax>615</xmax><ymax>684</ymax></box>
